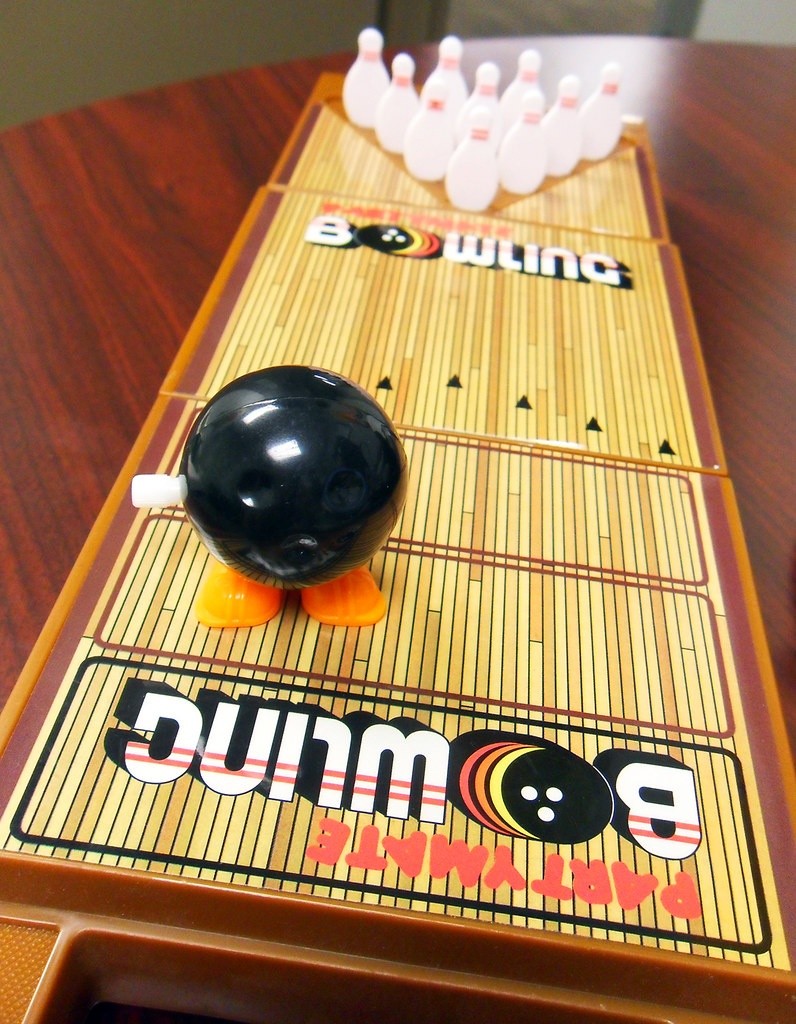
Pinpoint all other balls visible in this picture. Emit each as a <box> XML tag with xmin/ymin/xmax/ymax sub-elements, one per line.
<box><xmin>178</xmin><ymin>363</ymin><xmax>410</xmax><ymax>591</ymax></box>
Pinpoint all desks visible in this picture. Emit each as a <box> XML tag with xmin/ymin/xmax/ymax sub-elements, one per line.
<box><xmin>0</xmin><ymin>35</ymin><xmax>796</xmax><ymax>1023</ymax></box>
